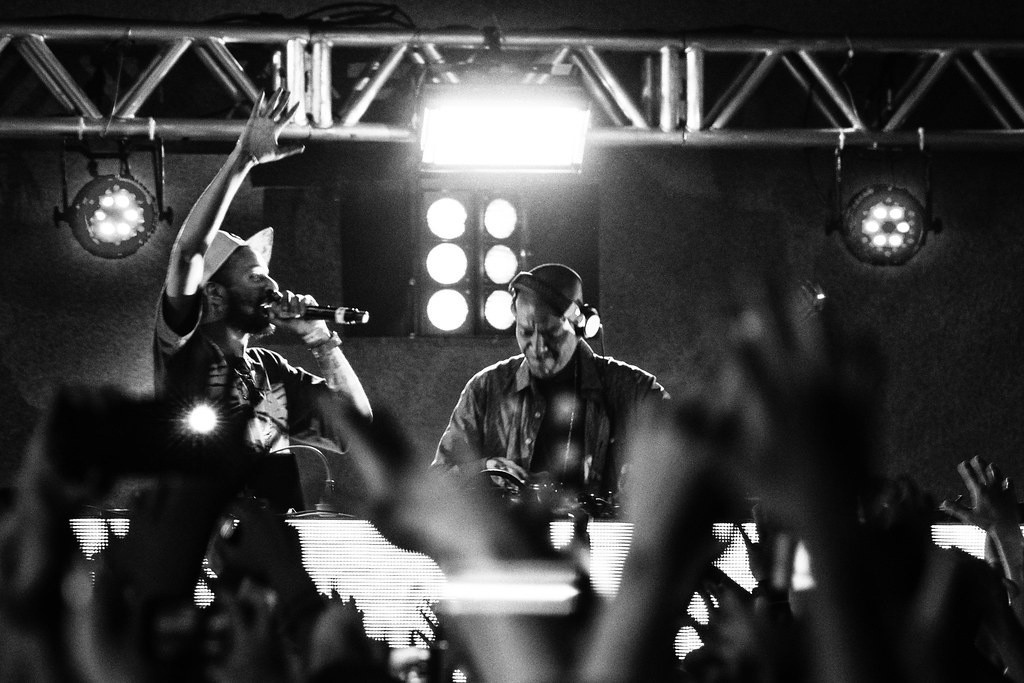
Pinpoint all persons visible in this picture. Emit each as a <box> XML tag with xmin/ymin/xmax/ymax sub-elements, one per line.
<box><xmin>151</xmin><ymin>84</ymin><xmax>376</xmax><ymax>512</ymax></box>
<box><xmin>0</xmin><ymin>270</ymin><xmax>1024</xmax><ymax>682</ymax></box>
<box><xmin>425</xmin><ymin>262</ymin><xmax>677</xmax><ymax>517</ymax></box>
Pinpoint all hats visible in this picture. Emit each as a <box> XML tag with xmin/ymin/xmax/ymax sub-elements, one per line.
<box><xmin>198</xmin><ymin>226</ymin><xmax>275</xmax><ymax>288</ymax></box>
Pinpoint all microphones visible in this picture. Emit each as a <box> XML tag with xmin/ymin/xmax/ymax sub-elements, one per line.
<box><xmin>272</xmin><ymin>299</ymin><xmax>370</xmax><ymax>324</ymax></box>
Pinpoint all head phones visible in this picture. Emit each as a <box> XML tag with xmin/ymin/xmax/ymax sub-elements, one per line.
<box><xmin>508</xmin><ymin>271</ymin><xmax>601</xmax><ymax>338</ymax></box>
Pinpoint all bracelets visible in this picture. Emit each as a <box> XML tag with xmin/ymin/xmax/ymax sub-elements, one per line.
<box><xmin>307</xmin><ymin>330</ymin><xmax>343</xmax><ymax>360</ymax></box>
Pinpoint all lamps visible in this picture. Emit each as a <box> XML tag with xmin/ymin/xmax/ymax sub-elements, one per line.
<box><xmin>345</xmin><ymin>181</ymin><xmax>527</xmax><ymax>339</ymax></box>
<box><xmin>819</xmin><ymin>143</ymin><xmax>946</xmax><ymax>266</ymax></box>
<box><xmin>51</xmin><ymin>145</ymin><xmax>173</xmax><ymax>259</ymax></box>
<box><xmin>410</xmin><ymin>52</ymin><xmax>590</xmax><ymax>176</ymax></box>
